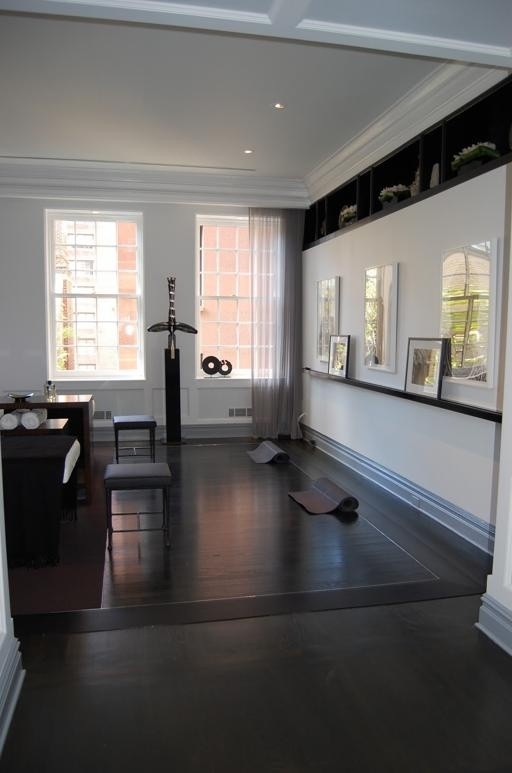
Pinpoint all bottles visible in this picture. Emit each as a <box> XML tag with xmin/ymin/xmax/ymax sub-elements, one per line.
<box><xmin>44</xmin><ymin>380</ymin><xmax>56</xmax><ymax>401</ymax></box>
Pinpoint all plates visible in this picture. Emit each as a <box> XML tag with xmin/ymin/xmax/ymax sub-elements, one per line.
<box><xmin>8</xmin><ymin>391</ymin><xmax>33</xmax><ymax>402</ymax></box>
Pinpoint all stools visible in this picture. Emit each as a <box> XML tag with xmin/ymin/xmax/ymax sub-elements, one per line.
<box><xmin>111</xmin><ymin>412</ymin><xmax>158</xmax><ymax>464</ymax></box>
<box><xmin>101</xmin><ymin>460</ymin><xmax>172</xmax><ymax>551</ymax></box>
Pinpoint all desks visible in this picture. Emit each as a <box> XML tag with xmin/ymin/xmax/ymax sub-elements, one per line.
<box><xmin>0</xmin><ymin>414</ymin><xmax>78</xmax><ymax>572</ymax></box>
<box><xmin>0</xmin><ymin>390</ymin><xmax>95</xmax><ymax>493</ymax></box>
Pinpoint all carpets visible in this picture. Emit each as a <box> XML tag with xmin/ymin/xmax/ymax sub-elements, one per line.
<box><xmin>14</xmin><ymin>436</ymin><xmax>492</xmax><ymax>636</ymax></box>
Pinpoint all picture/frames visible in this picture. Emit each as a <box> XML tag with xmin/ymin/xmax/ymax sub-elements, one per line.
<box><xmin>440</xmin><ymin>236</ymin><xmax>500</xmax><ymax>390</ymax></box>
<box><xmin>362</xmin><ymin>260</ymin><xmax>398</xmax><ymax>374</ymax></box>
<box><xmin>328</xmin><ymin>333</ymin><xmax>352</xmax><ymax>378</ymax></box>
<box><xmin>315</xmin><ymin>274</ymin><xmax>341</xmax><ymax>363</ymax></box>
<box><xmin>404</xmin><ymin>335</ymin><xmax>449</xmax><ymax>400</ymax></box>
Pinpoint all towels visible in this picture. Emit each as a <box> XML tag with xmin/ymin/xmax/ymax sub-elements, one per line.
<box><xmin>21</xmin><ymin>407</ymin><xmax>49</xmax><ymax>429</ymax></box>
<box><xmin>0</xmin><ymin>406</ymin><xmax>28</xmax><ymax>430</ymax></box>
